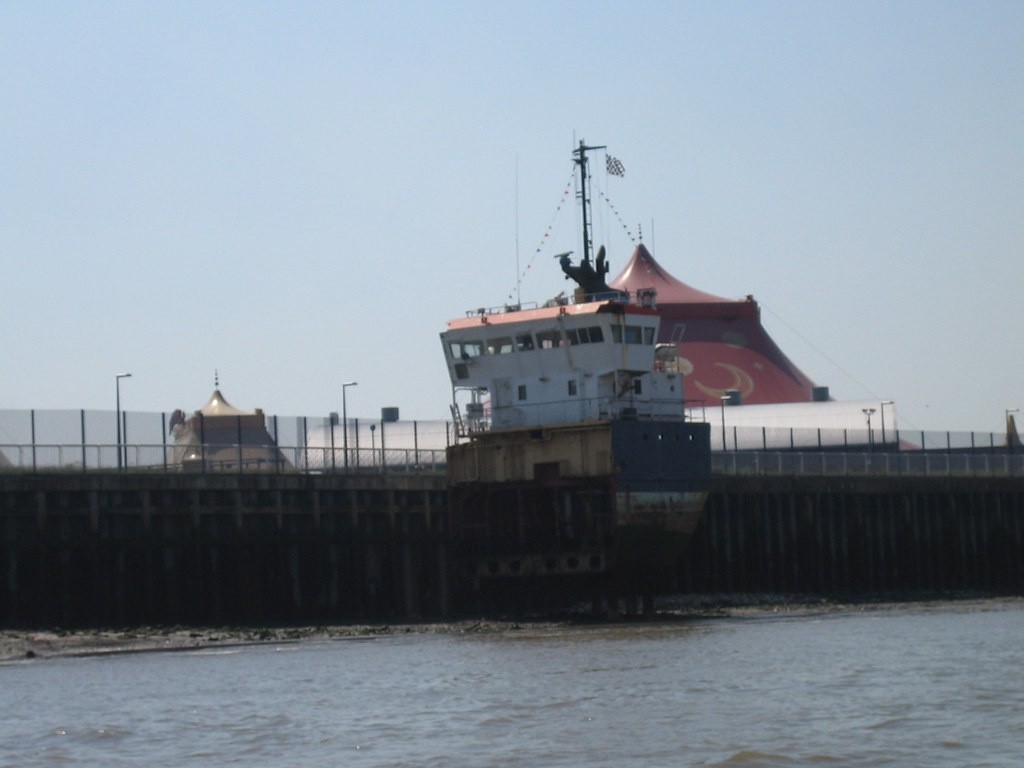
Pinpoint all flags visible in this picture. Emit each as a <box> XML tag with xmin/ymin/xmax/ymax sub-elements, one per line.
<box><xmin>606</xmin><ymin>154</ymin><xmax>625</xmax><ymax>177</ymax></box>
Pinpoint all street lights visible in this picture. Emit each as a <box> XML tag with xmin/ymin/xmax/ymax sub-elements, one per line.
<box><xmin>342</xmin><ymin>382</ymin><xmax>357</xmax><ymax>470</ymax></box>
<box><xmin>881</xmin><ymin>401</ymin><xmax>894</xmax><ymax>443</ymax></box>
<box><xmin>115</xmin><ymin>374</ymin><xmax>132</xmax><ymax>472</ymax></box>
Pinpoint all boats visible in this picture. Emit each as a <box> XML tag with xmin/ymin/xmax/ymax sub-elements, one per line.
<box><xmin>436</xmin><ymin>135</ymin><xmax>711</xmax><ymax>489</ymax></box>
